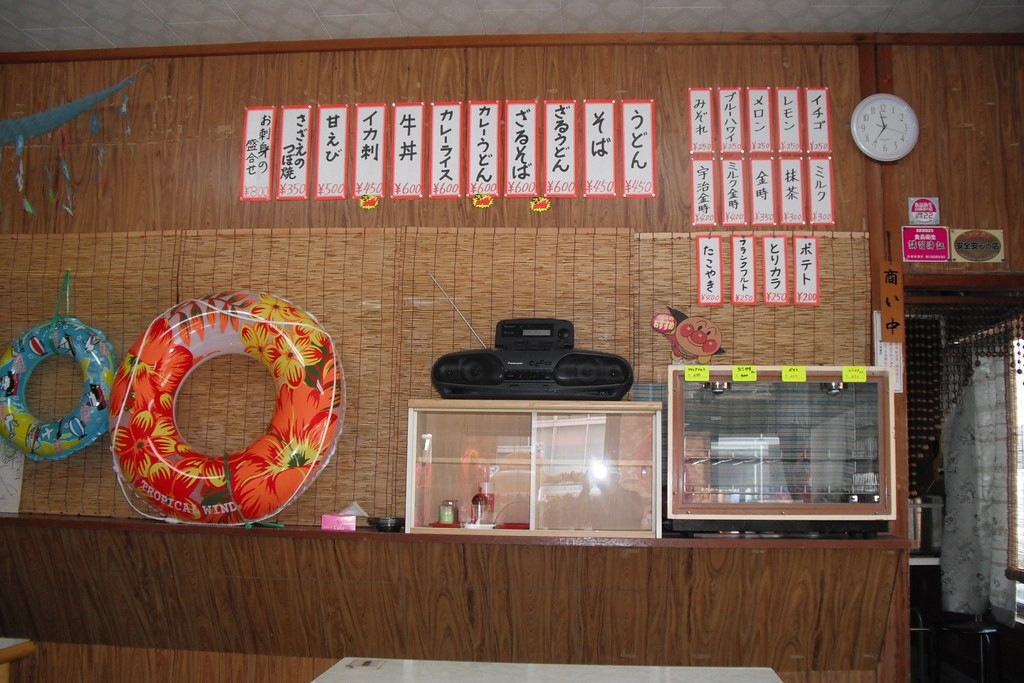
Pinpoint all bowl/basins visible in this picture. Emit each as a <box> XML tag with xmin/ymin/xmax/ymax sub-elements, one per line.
<box><xmin>368</xmin><ymin>517</ymin><xmax>405</xmax><ymax>532</ymax></box>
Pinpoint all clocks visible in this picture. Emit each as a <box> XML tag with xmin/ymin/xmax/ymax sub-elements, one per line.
<box><xmin>851</xmin><ymin>92</ymin><xmax>920</xmax><ymax>161</ymax></box>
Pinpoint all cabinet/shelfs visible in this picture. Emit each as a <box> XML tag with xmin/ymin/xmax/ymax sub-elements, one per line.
<box><xmin>407</xmin><ymin>399</ymin><xmax>663</xmax><ymax>538</ymax></box>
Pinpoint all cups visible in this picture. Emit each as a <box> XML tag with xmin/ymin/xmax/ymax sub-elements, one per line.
<box><xmin>479</xmin><ymin>482</ymin><xmax>495</xmax><ymax>523</ymax></box>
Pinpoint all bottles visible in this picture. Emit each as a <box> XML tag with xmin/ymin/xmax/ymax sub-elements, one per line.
<box><xmin>471</xmin><ymin>486</ymin><xmax>490</xmax><ymax>524</ymax></box>
<box><xmin>442</xmin><ymin>499</ymin><xmax>459</xmax><ymax>523</ymax></box>
<box><xmin>438</xmin><ymin>505</ymin><xmax>454</xmax><ymax>525</ymax></box>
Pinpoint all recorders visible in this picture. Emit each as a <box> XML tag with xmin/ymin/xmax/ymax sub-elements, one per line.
<box><xmin>425</xmin><ymin>269</ymin><xmax>633</xmax><ymax>402</ymax></box>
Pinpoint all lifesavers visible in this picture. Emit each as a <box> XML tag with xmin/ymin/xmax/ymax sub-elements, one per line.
<box><xmin>0</xmin><ymin>319</ymin><xmax>120</xmax><ymax>462</ymax></box>
<box><xmin>107</xmin><ymin>289</ymin><xmax>345</xmax><ymax>526</ymax></box>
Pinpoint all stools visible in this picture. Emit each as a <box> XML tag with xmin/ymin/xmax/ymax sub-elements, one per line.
<box><xmin>911</xmin><ymin>598</ymin><xmax>1004</xmax><ymax>683</ymax></box>
